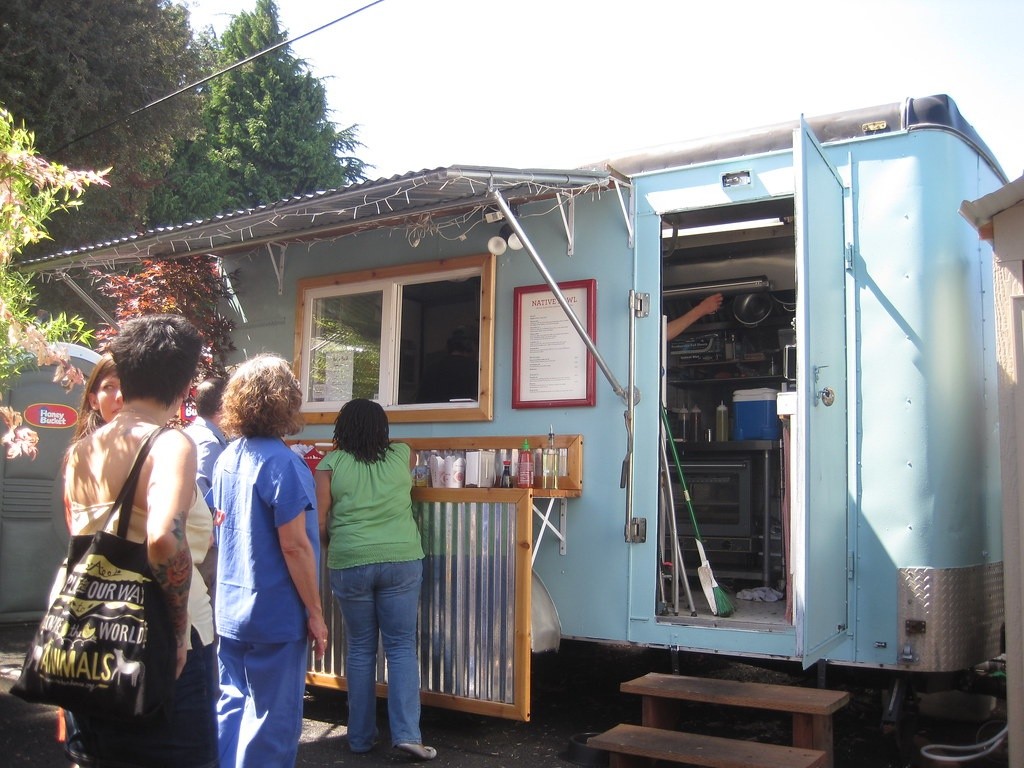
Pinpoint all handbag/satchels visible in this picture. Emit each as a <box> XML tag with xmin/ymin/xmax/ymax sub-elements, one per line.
<box><xmin>6</xmin><ymin>531</ymin><xmax>173</xmax><ymax>720</ymax></box>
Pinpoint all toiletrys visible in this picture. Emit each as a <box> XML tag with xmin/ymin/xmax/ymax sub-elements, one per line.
<box><xmin>406</xmin><ymin>422</ymin><xmax>562</xmax><ymax>491</ymax></box>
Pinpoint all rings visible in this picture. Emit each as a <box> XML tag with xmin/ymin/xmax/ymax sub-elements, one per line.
<box><xmin>322</xmin><ymin>639</ymin><xmax>327</xmax><ymax>643</ymax></box>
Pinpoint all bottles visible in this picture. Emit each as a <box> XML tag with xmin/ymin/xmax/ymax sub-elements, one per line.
<box><xmin>410</xmin><ymin>465</ymin><xmax>417</xmax><ymax>487</ymax></box>
<box><xmin>500</xmin><ymin>460</ymin><xmax>514</xmax><ymax>489</ymax></box>
<box><xmin>715</xmin><ymin>400</ymin><xmax>729</xmax><ymax>442</ymax></box>
<box><xmin>542</xmin><ymin>425</ymin><xmax>559</xmax><ymax>490</ymax></box>
<box><xmin>426</xmin><ymin>449</ymin><xmax>439</xmax><ymax>487</ymax></box>
<box><xmin>677</xmin><ymin>404</ymin><xmax>690</xmax><ymax>442</ymax></box>
<box><xmin>516</xmin><ymin>438</ymin><xmax>535</xmax><ymax>488</ymax></box>
<box><xmin>415</xmin><ymin>449</ymin><xmax>428</xmax><ymax>488</ymax></box>
<box><xmin>690</xmin><ymin>403</ymin><xmax>702</xmax><ymax>442</ymax></box>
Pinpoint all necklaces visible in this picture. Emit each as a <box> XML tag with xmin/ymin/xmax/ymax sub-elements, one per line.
<box><xmin>115</xmin><ymin>406</ymin><xmax>160</xmax><ymax>427</ymax></box>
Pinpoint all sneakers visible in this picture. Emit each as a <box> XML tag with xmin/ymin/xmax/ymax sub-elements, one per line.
<box><xmin>397</xmin><ymin>742</ymin><xmax>437</xmax><ymax>760</ymax></box>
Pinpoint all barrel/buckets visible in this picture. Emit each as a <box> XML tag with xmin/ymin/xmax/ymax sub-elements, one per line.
<box><xmin>732</xmin><ymin>388</ymin><xmax>781</xmax><ymax>442</ymax></box>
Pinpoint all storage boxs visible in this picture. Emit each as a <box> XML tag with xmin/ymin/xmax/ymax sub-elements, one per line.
<box><xmin>731</xmin><ymin>387</ymin><xmax>782</xmax><ymax>441</ymax></box>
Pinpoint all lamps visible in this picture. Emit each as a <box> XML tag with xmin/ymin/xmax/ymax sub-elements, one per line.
<box><xmin>488</xmin><ymin>224</ymin><xmax>524</xmax><ymax>255</ymax></box>
<box><xmin>663</xmin><ymin>216</ymin><xmax>788</xmax><ymax>239</ymax></box>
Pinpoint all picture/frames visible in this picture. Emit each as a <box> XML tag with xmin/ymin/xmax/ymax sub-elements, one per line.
<box><xmin>512</xmin><ymin>279</ymin><xmax>596</xmax><ymax>409</ymax></box>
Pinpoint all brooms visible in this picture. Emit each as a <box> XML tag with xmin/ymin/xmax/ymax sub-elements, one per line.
<box><xmin>662</xmin><ymin>394</ymin><xmax>741</xmax><ymax>616</ymax></box>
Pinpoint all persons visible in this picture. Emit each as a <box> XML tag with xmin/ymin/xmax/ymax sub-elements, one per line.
<box><xmin>61</xmin><ymin>316</ymin><xmax>221</xmax><ymax>768</ymax></box>
<box><xmin>312</xmin><ymin>398</ymin><xmax>444</xmax><ymax>762</ymax></box>
<box><xmin>213</xmin><ymin>352</ymin><xmax>327</xmax><ymax>768</ymax></box>
<box><xmin>72</xmin><ymin>354</ymin><xmax>130</xmax><ymax>443</ymax></box>
<box><xmin>177</xmin><ymin>376</ymin><xmax>234</xmax><ymax>619</ymax></box>
<box><xmin>661</xmin><ymin>294</ymin><xmax>725</xmax><ymax>348</ymax></box>
